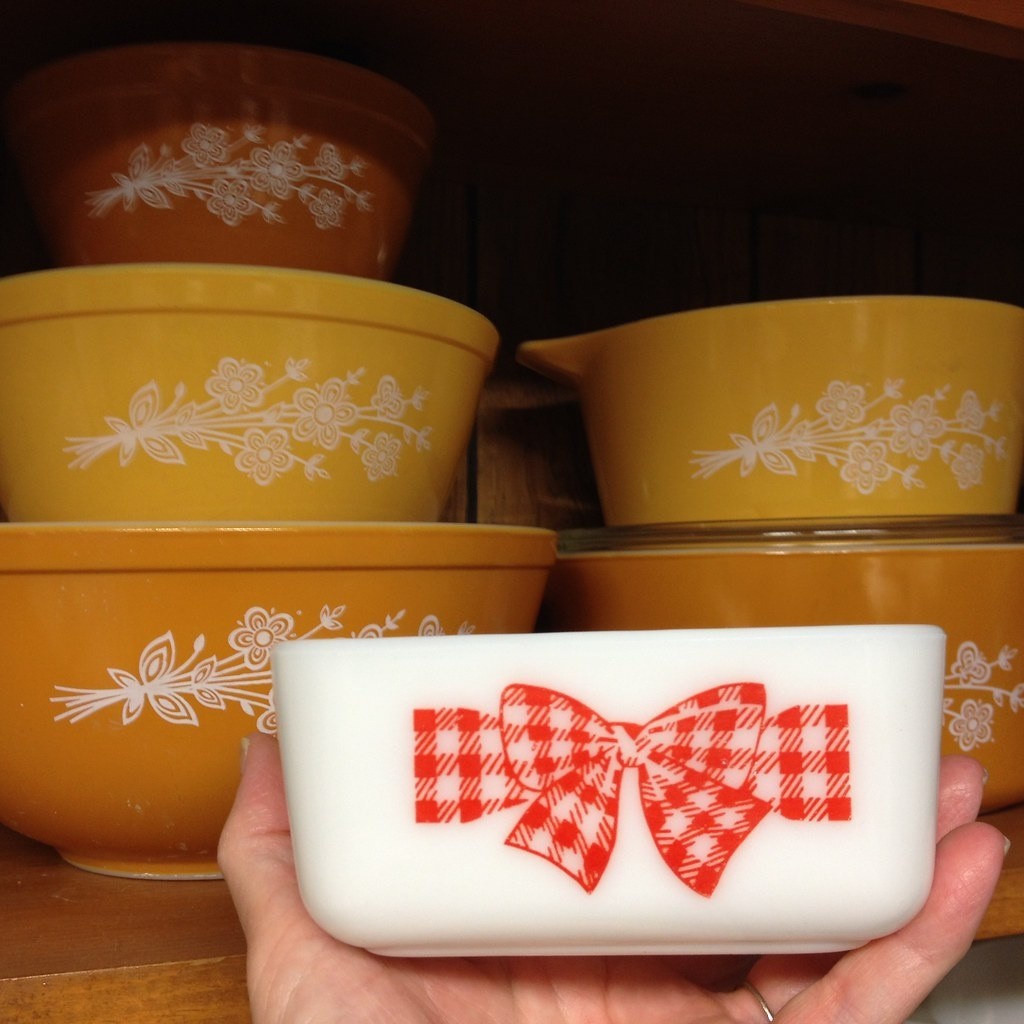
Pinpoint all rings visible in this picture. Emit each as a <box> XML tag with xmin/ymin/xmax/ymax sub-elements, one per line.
<box><xmin>741</xmin><ymin>980</ymin><xmax>774</xmax><ymax>1023</ymax></box>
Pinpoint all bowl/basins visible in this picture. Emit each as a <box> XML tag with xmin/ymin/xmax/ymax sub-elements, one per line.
<box><xmin>0</xmin><ymin>40</ymin><xmax>436</xmax><ymax>289</ymax></box>
<box><xmin>0</xmin><ymin>252</ymin><xmax>1024</xmax><ymax>965</ymax></box>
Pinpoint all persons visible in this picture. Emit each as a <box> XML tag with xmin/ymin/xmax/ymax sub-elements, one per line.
<box><xmin>217</xmin><ymin>732</ymin><xmax>1012</xmax><ymax>1024</ymax></box>
